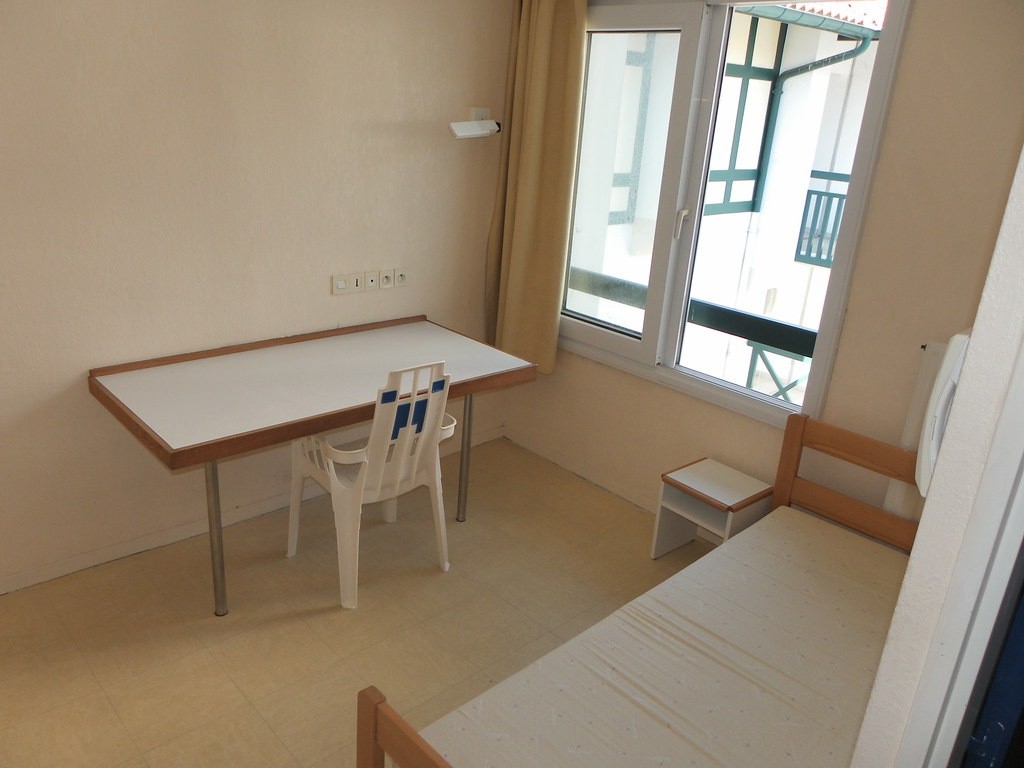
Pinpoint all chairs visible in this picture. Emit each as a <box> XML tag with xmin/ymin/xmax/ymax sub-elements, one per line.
<box><xmin>286</xmin><ymin>361</ymin><xmax>457</xmax><ymax>609</ymax></box>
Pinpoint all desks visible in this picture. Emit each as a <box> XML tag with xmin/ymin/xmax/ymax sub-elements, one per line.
<box><xmin>651</xmin><ymin>456</ymin><xmax>774</xmax><ymax>559</ymax></box>
<box><xmin>88</xmin><ymin>315</ymin><xmax>538</xmax><ymax>617</ymax></box>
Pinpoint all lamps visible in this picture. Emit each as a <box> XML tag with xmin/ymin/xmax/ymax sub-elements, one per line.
<box><xmin>450</xmin><ymin>119</ymin><xmax>501</xmax><ymax>139</ymax></box>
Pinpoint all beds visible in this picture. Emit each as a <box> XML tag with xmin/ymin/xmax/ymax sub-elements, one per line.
<box><xmin>357</xmin><ymin>413</ymin><xmax>919</xmax><ymax>768</ymax></box>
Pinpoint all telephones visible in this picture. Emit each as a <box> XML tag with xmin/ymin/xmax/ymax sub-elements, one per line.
<box><xmin>915</xmin><ymin>333</ymin><xmax>972</xmax><ymax>498</ymax></box>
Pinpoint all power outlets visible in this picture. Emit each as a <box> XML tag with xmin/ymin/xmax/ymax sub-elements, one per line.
<box><xmin>472</xmin><ymin>108</ymin><xmax>491</xmax><ymax>121</ymax></box>
